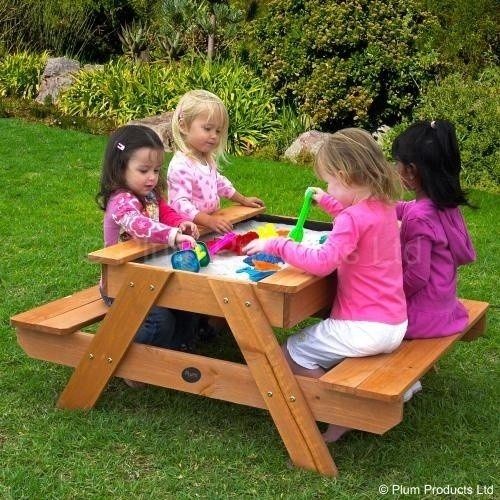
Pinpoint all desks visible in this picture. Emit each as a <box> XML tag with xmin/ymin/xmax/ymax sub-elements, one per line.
<box><xmin>55</xmin><ymin>205</ymin><xmax>339</xmax><ymax>482</ymax></box>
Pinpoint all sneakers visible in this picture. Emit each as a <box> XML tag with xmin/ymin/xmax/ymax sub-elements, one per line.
<box><xmin>403</xmin><ymin>389</ymin><xmax>412</xmax><ymax>402</ymax></box>
<box><xmin>412</xmin><ymin>380</ymin><xmax>421</xmax><ymax>395</ymax></box>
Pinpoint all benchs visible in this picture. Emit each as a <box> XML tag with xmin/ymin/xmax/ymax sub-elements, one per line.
<box><xmin>10</xmin><ymin>279</ymin><xmax>119</xmax><ymax>367</ymax></box>
<box><xmin>317</xmin><ymin>296</ymin><xmax>491</xmax><ymax>434</ymax></box>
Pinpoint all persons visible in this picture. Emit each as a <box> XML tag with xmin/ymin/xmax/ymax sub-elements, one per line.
<box><xmin>240</xmin><ymin>125</ymin><xmax>413</xmax><ymax>449</ymax></box>
<box><xmin>289</xmin><ymin>188</ymin><xmax>316</xmax><ymax>244</ymax></box>
<box><xmin>388</xmin><ymin>116</ymin><xmax>479</xmax><ymax>406</ymax></box>
<box><xmin>96</xmin><ymin>123</ymin><xmax>201</xmax><ymax>390</ymax></box>
<box><xmin>171</xmin><ymin>222</ymin><xmax>292</xmax><ymax>282</ymax></box>
<box><xmin>165</xmin><ymin>88</ymin><xmax>266</xmax><ymax>352</ymax></box>
